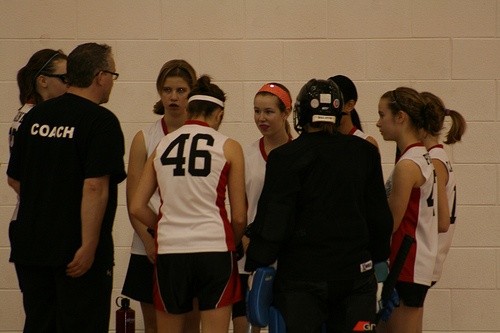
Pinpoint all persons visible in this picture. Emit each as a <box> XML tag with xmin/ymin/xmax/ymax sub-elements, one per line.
<box><xmin>231</xmin><ymin>81</ymin><xmax>297</xmax><ymax>333</ymax></box>
<box><xmin>327</xmin><ymin>74</ymin><xmax>379</xmax><ymax>150</ymax></box>
<box><xmin>7</xmin><ymin>42</ymin><xmax>126</xmax><ymax>332</ymax></box>
<box><xmin>417</xmin><ymin>91</ymin><xmax>467</xmax><ymax>333</ymax></box>
<box><xmin>376</xmin><ymin>86</ymin><xmax>439</xmax><ymax>333</ymax></box>
<box><xmin>9</xmin><ymin>48</ymin><xmax>69</xmax><ymax>333</ymax></box>
<box><xmin>127</xmin><ymin>75</ymin><xmax>248</xmax><ymax>333</ymax></box>
<box><xmin>242</xmin><ymin>78</ymin><xmax>395</xmax><ymax>333</ymax></box>
<box><xmin>121</xmin><ymin>59</ymin><xmax>198</xmax><ymax>333</ymax></box>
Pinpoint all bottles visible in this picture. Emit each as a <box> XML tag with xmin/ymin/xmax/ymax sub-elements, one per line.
<box><xmin>116</xmin><ymin>296</ymin><xmax>136</xmax><ymax>333</ymax></box>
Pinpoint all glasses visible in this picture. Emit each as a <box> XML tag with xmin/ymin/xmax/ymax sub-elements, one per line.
<box><xmin>41</xmin><ymin>72</ymin><xmax>68</xmax><ymax>84</ymax></box>
<box><xmin>93</xmin><ymin>69</ymin><xmax>119</xmax><ymax>81</ymax></box>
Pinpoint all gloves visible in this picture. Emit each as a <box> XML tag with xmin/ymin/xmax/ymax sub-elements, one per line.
<box><xmin>375</xmin><ymin>263</ymin><xmax>399</xmax><ymax>318</ymax></box>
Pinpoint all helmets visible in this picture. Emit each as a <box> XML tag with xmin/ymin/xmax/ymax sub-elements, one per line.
<box><xmin>292</xmin><ymin>78</ymin><xmax>345</xmax><ymax>134</ymax></box>
